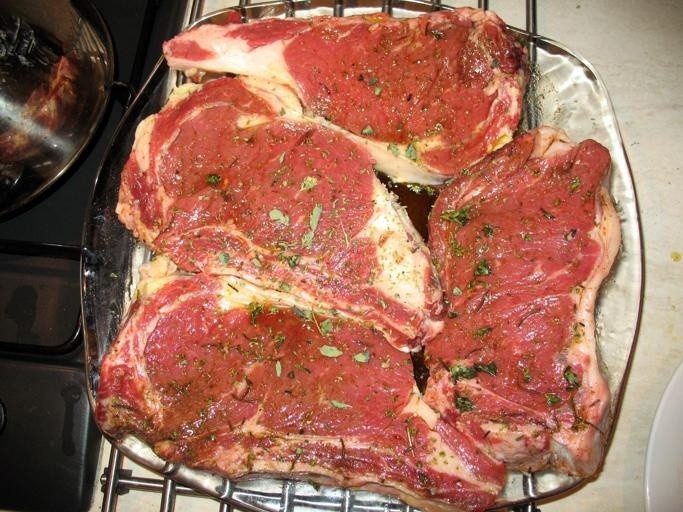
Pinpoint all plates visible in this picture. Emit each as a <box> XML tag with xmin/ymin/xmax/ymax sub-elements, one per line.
<box><xmin>644</xmin><ymin>360</ymin><xmax>683</xmax><ymax>512</ymax></box>
